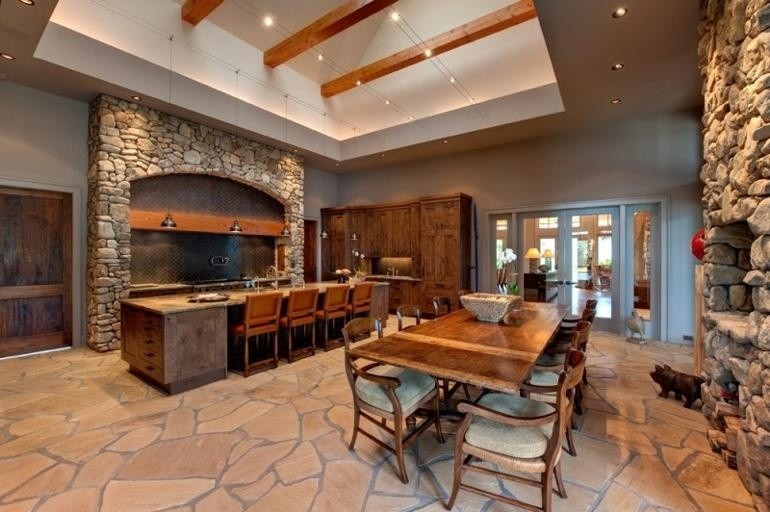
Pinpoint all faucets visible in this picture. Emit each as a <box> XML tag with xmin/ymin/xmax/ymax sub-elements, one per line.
<box><xmin>266</xmin><ymin>264</ymin><xmax>280</xmax><ymax>291</ymax></box>
<box><xmin>388</xmin><ymin>266</ymin><xmax>395</xmax><ymax>276</ymax></box>
<box><xmin>252</xmin><ymin>274</ymin><xmax>261</xmax><ymax>293</ymax></box>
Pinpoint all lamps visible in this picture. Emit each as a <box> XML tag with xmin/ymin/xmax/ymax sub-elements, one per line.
<box><xmin>542</xmin><ymin>249</ymin><xmax>555</xmax><ymax>258</ymax></box>
<box><xmin>351</xmin><ymin>126</ymin><xmax>360</xmax><ymax>240</ymax></box>
<box><xmin>229</xmin><ymin>69</ymin><xmax>243</xmax><ymax>232</ymax></box>
<box><xmin>160</xmin><ymin>34</ymin><xmax>178</xmax><ymax>229</ymax></box>
<box><xmin>281</xmin><ymin>93</ymin><xmax>291</xmax><ymax>236</ymax></box>
<box><xmin>523</xmin><ymin>248</ymin><xmax>541</xmax><ymax>258</ymax></box>
<box><xmin>320</xmin><ymin>111</ymin><xmax>329</xmax><ymax>239</ymax></box>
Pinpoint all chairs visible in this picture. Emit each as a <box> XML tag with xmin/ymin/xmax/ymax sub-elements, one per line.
<box><xmin>226</xmin><ymin>292</ymin><xmax>284</xmax><ymax>378</ymax></box>
<box><xmin>347</xmin><ymin>282</ymin><xmax>375</xmax><ymax>342</ymax></box>
<box><xmin>277</xmin><ymin>288</ymin><xmax>320</xmax><ymax>363</ymax></box>
<box><xmin>342</xmin><ymin>291</ymin><xmax>599</xmax><ymax>512</ymax></box>
<box><xmin>315</xmin><ymin>284</ymin><xmax>351</xmax><ymax>352</ymax></box>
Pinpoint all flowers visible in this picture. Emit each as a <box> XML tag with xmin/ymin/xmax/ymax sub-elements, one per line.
<box><xmin>494</xmin><ymin>246</ymin><xmax>520</xmax><ymax>295</ymax></box>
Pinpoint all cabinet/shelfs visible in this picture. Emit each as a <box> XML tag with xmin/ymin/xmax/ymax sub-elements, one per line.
<box><xmin>393</xmin><ymin>204</ymin><xmax>420</xmax><ymax>257</ymax></box>
<box><xmin>422</xmin><ymin>280</ymin><xmax>471</xmax><ymax>316</ymax></box>
<box><xmin>321</xmin><ymin>210</ymin><xmax>345</xmax><ymax>280</ymax></box>
<box><xmin>388</xmin><ymin>280</ymin><xmax>422</xmax><ymax>313</ymax></box>
<box><xmin>419</xmin><ymin>198</ymin><xmax>472</xmax><ymax>280</ymax></box>
<box><xmin>368</xmin><ymin>210</ymin><xmax>393</xmax><ymax>257</ymax></box>
<box><xmin>524</xmin><ymin>270</ymin><xmax>559</xmax><ymax>303</ymax></box>
<box><xmin>345</xmin><ymin>211</ymin><xmax>367</xmax><ymax>258</ymax></box>
